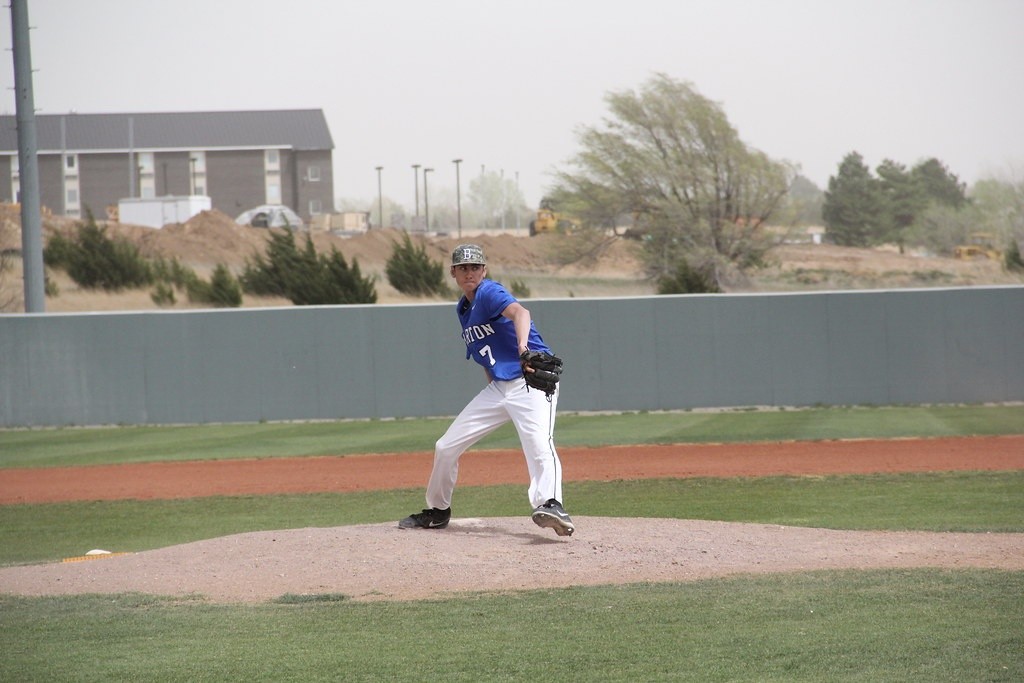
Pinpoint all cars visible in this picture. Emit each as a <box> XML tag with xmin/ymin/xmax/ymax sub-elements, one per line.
<box><xmin>236</xmin><ymin>205</ymin><xmax>304</xmax><ymax>233</ymax></box>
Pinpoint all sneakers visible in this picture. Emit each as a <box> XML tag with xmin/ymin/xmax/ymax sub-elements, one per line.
<box><xmin>532</xmin><ymin>498</ymin><xmax>574</xmax><ymax>536</ymax></box>
<box><xmin>398</xmin><ymin>507</ymin><xmax>451</xmax><ymax>529</ymax></box>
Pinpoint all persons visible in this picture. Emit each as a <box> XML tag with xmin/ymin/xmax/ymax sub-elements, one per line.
<box><xmin>398</xmin><ymin>244</ymin><xmax>574</xmax><ymax>536</ymax></box>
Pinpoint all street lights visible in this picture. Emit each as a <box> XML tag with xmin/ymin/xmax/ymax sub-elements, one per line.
<box><xmin>452</xmin><ymin>159</ymin><xmax>463</xmax><ymax>238</ymax></box>
<box><xmin>412</xmin><ymin>165</ymin><xmax>421</xmax><ymax>215</ymax></box>
<box><xmin>375</xmin><ymin>165</ymin><xmax>384</xmax><ymax>228</ymax></box>
<box><xmin>424</xmin><ymin>168</ymin><xmax>435</xmax><ymax>231</ymax></box>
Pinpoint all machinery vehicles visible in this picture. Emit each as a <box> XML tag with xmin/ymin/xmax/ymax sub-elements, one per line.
<box><xmin>529</xmin><ymin>207</ymin><xmax>583</xmax><ymax>236</ymax></box>
<box><xmin>950</xmin><ymin>232</ymin><xmax>1008</xmax><ymax>269</ymax></box>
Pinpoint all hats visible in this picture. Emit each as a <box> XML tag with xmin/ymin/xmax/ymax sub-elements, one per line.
<box><xmin>451</xmin><ymin>244</ymin><xmax>486</xmax><ymax>266</ymax></box>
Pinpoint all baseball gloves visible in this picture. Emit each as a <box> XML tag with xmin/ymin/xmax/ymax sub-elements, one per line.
<box><xmin>519</xmin><ymin>345</ymin><xmax>564</xmax><ymax>402</ymax></box>
